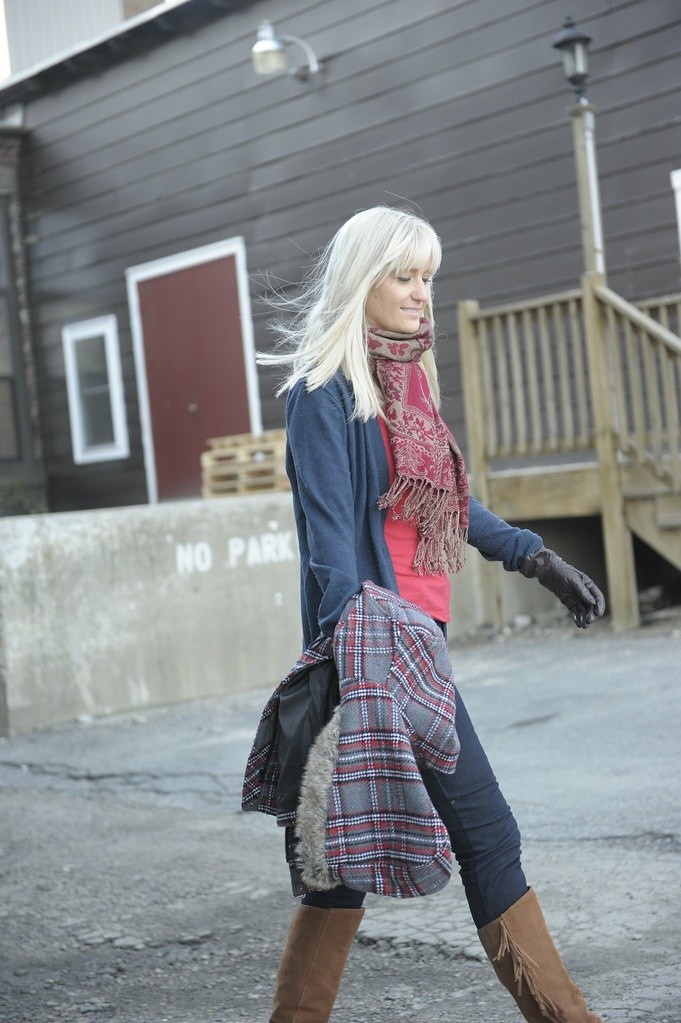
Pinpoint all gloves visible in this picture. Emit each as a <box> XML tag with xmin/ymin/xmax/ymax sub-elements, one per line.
<box><xmin>519</xmin><ymin>544</ymin><xmax>605</xmax><ymax>629</ymax></box>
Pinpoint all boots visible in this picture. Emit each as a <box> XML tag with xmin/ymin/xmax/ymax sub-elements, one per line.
<box><xmin>268</xmin><ymin>904</ymin><xmax>365</xmax><ymax>1023</ymax></box>
<box><xmin>477</xmin><ymin>886</ymin><xmax>605</xmax><ymax>1023</ymax></box>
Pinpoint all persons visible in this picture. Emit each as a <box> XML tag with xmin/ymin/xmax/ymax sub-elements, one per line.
<box><xmin>267</xmin><ymin>206</ymin><xmax>605</xmax><ymax>1023</ymax></box>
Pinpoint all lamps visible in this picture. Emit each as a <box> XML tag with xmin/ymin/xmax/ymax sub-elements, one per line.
<box><xmin>251</xmin><ymin>18</ymin><xmax>323</xmax><ymax>84</ymax></box>
<box><xmin>550</xmin><ymin>14</ymin><xmax>593</xmax><ymax>105</ymax></box>
